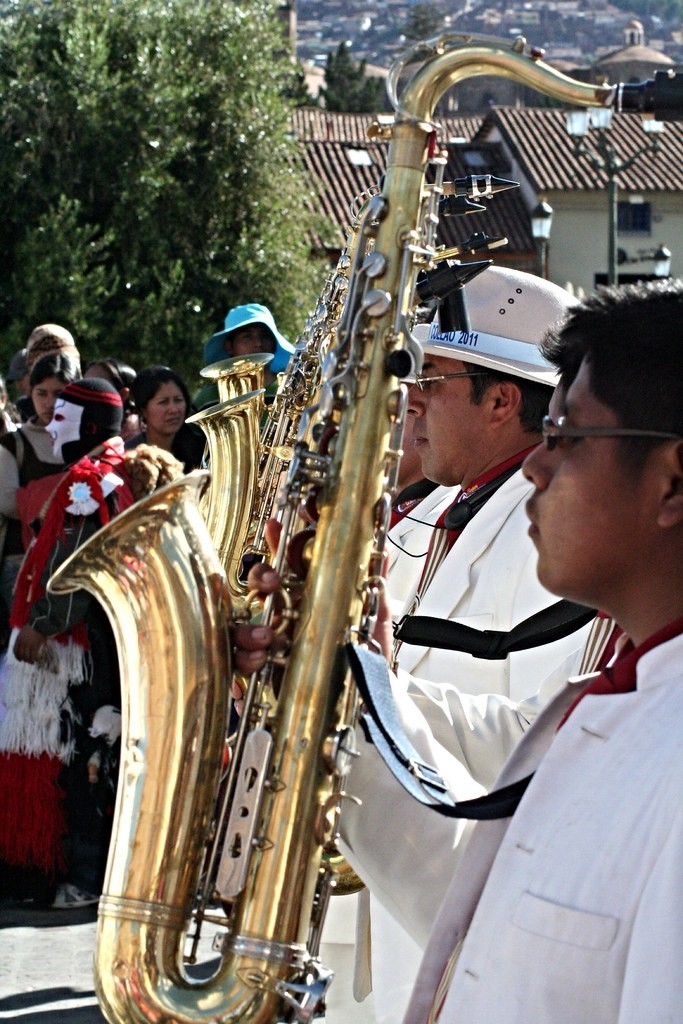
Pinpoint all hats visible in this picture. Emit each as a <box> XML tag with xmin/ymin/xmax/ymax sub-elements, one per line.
<box><xmin>6</xmin><ymin>348</ymin><xmax>30</xmax><ymax>382</ymax></box>
<box><xmin>27</xmin><ymin>323</ymin><xmax>75</xmax><ymax>366</ymax></box>
<box><xmin>52</xmin><ymin>376</ymin><xmax>124</xmax><ymax>466</ymax></box>
<box><xmin>204</xmin><ymin>303</ymin><xmax>296</xmax><ymax>374</ymax></box>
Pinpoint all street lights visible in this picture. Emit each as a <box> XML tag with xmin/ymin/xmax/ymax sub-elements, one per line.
<box><xmin>560</xmin><ymin>75</ymin><xmax>669</xmax><ymax>287</ymax></box>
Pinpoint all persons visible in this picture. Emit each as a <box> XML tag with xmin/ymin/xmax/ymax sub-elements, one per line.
<box><xmin>0</xmin><ymin>303</ymin><xmax>297</xmax><ymax>910</ymax></box>
<box><xmin>351</xmin><ymin>263</ymin><xmax>598</xmax><ymax>1024</ymax></box>
<box><xmin>379</xmin><ymin>323</ymin><xmax>462</xmax><ymax>653</ymax></box>
<box><xmin>236</xmin><ymin>282</ymin><xmax>683</xmax><ymax>1024</ymax></box>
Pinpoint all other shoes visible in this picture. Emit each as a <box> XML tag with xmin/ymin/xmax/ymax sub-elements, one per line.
<box><xmin>51</xmin><ymin>884</ymin><xmax>100</xmax><ymax>908</ymax></box>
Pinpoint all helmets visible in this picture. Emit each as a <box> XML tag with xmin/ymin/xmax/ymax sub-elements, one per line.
<box><xmin>412</xmin><ymin>264</ymin><xmax>586</xmax><ymax>386</ymax></box>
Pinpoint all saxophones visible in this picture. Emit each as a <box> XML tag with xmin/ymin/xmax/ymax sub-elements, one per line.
<box><xmin>50</xmin><ymin>46</ymin><xmax>682</xmax><ymax>1024</ymax></box>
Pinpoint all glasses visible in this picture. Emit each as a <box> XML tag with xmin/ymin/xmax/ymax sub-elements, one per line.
<box><xmin>540</xmin><ymin>415</ymin><xmax>678</xmax><ymax>452</ymax></box>
<box><xmin>414</xmin><ymin>371</ymin><xmax>530</xmax><ymax>396</ymax></box>
